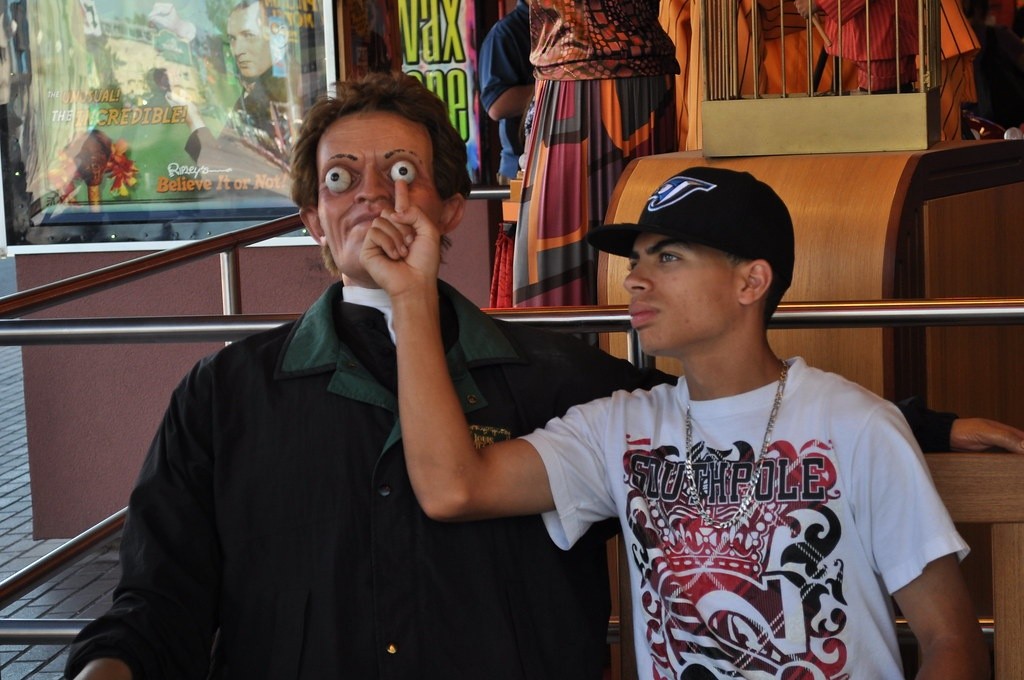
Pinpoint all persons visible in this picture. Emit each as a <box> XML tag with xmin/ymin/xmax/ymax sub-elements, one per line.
<box><xmin>513</xmin><ymin>0</ymin><xmax>681</xmax><ymax>347</ymax></box>
<box><xmin>358</xmin><ymin>166</ymin><xmax>991</xmax><ymax>680</ymax></box>
<box><xmin>64</xmin><ymin>73</ymin><xmax>1024</xmax><ymax>680</ymax></box>
<box><xmin>791</xmin><ymin>0</ymin><xmax>927</xmax><ymax>93</ymax></box>
<box><xmin>164</xmin><ymin>0</ymin><xmax>304</xmax><ymax>197</ymax></box>
<box><xmin>478</xmin><ymin>0</ymin><xmax>535</xmax><ymax>236</ymax></box>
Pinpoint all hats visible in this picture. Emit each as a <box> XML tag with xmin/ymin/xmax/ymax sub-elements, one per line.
<box><xmin>587</xmin><ymin>167</ymin><xmax>795</xmax><ymax>290</ymax></box>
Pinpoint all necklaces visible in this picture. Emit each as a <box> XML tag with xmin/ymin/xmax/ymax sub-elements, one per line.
<box><xmin>685</xmin><ymin>359</ymin><xmax>788</xmax><ymax>529</ymax></box>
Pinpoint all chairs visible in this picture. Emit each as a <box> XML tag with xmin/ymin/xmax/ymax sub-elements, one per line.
<box><xmin>615</xmin><ymin>450</ymin><xmax>1024</xmax><ymax>680</ymax></box>
